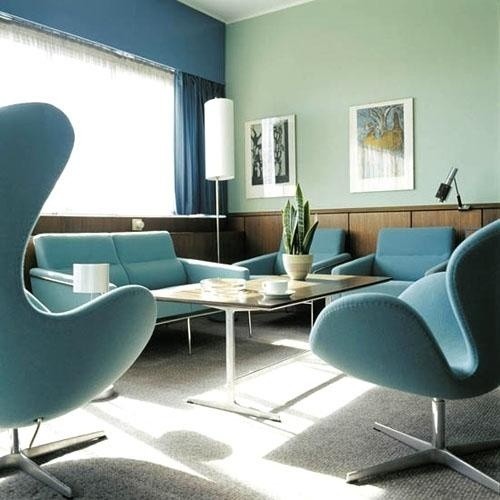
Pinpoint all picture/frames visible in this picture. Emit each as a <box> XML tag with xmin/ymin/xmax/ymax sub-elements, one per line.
<box><xmin>349</xmin><ymin>97</ymin><xmax>415</xmax><ymax>193</ymax></box>
<box><xmin>245</xmin><ymin>114</ymin><xmax>297</xmax><ymax>199</ymax></box>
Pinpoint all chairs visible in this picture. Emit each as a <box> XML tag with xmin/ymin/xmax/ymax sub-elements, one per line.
<box><xmin>232</xmin><ymin>226</ymin><xmax>352</xmax><ymax>330</ymax></box>
<box><xmin>0</xmin><ymin>102</ymin><xmax>157</xmax><ymax>500</ymax></box>
<box><xmin>331</xmin><ymin>227</ymin><xmax>457</xmax><ymax>302</ymax></box>
<box><xmin>310</xmin><ymin>219</ymin><xmax>500</xmax><ymax>500</ymax></box>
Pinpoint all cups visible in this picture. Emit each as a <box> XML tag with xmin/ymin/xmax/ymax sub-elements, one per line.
<box><xmin>262</xmin><ymin>280</ymin><xmax>288</xmax><ymax>294</ymax></box>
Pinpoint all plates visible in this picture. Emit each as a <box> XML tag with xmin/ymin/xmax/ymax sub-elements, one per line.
<box><xmin>258</xmin><ymin>289</ymin><xmax>296</xmax><ymax>299</ymax></box>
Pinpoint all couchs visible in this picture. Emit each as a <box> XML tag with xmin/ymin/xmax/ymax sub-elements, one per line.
<box><xmin>29</xmin><ymin>231</ymin><xmax>253</xmax><ymax>355</ymax></box>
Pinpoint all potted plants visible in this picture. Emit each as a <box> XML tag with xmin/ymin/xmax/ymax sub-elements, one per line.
<box><xmin>281</xmin><ymin>184</ymin><xmax>319</xmax><ymax>281</ymax></box>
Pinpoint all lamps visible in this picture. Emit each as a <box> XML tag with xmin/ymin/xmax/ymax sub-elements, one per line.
<box><xmin>436</xmin><ymin>167</ymin><xmax>470</xmax><ymax>210</ymax></box>
<box><xmin>203</xmin><ymin>96</ymin><xmax>236</xmax><ymax>262</ymax></box>
<box><xmin>72</xmin><ymin>263</ymin><xmax>115</xmax><ymax>399</ymax></box>
